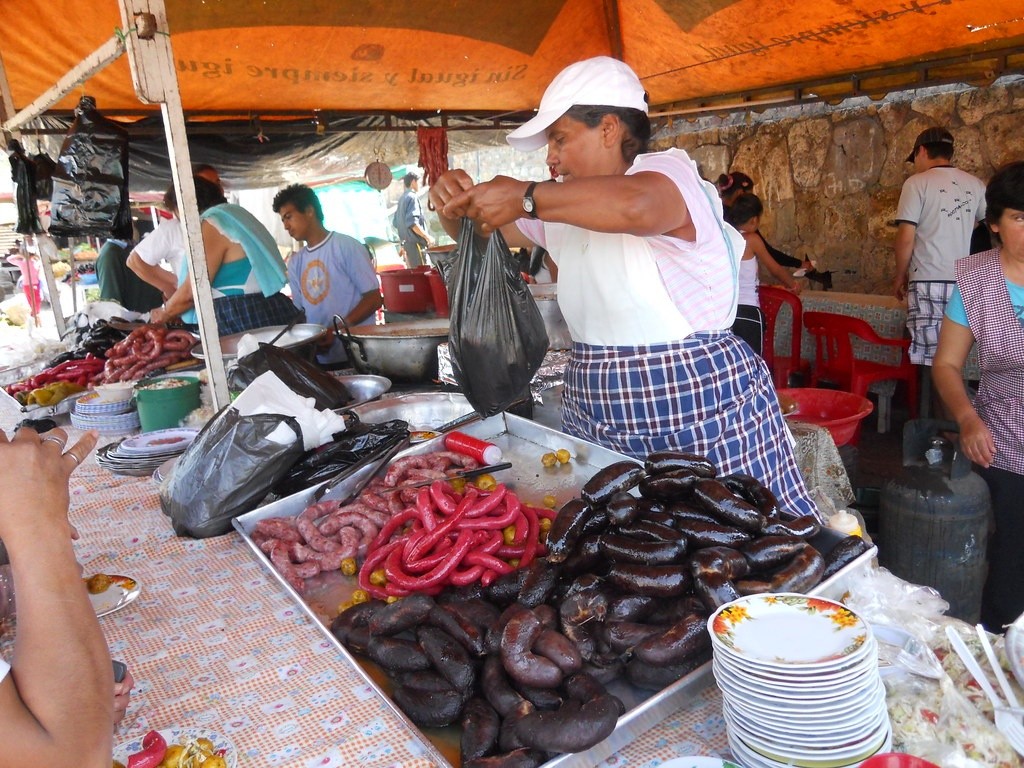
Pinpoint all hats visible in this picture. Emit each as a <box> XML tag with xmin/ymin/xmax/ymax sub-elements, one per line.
<box><xmin>903</xmin><ymin>128</ymin><xmax>954</xmax><ymax>164</ymax></box>
<box><xmin>506</xmin><ymin>55</ymin><xmax>650</xmax><ymax>152</ymax></box>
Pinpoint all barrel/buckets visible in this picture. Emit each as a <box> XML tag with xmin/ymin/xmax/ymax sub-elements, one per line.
<box><xmin>378</xmin><ymin>268</ymin><xmax>450</xmax><ymax>317</ymax></box>
<box><xmin>134</xmin><ymin>377</ymin><xmax>201</xmax><ymax>431</ymax></box>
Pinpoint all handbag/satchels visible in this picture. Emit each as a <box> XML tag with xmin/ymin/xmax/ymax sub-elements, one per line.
<box><xmin>156</xmin><ymin>406</ymin><xmax>305</xmax><ymax>538</ymax></box>
<box><xmin>237</xmin><ymin>344</ymin><xmax>351</xmax><ymax>415</ymax></box>
<box><xmin>445</xmin><ymin>217</ymin><xmax>549</xmax><ymax>422</ymax></box>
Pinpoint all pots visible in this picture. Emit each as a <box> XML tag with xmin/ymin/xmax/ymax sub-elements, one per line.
<box><xmin>331</xmin><ymin>315</ymin><xmax>453</xmax><ymax>380</ymax></box>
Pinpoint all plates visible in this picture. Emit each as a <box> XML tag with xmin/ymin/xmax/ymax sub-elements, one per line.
<box><xmin>95</xmin><ymin>428</ymin><xmax>201</xmax><ymax>484</ymax></box>
<box><xmin>112</xmin><ymin>728</ymin><xmax>236</xmax><ymax>768</ymax></box>
<box><xmin>83</xmin><ymin>573</ymin><xmax>142</xmax><ymax>618</ymax></box>
<box><xmin>70</xmin><ymin>392</ymin><xmax>140</xmax><ymax>434</ymax></box>
<box><xmin>408</xmin><ymin>431</ymin><xmax>445</xmax><ymax>444</ymax></box>
<box><xmin>656</xmin><ymin>592</ymin><xmax>941</xmax><ymax>768</ymax></box>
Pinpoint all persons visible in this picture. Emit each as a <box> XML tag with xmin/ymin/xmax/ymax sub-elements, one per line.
<box><xmin>889</xmin><ymin>125</ymin><xmax>985</xmax><ymax>450</ymax></box>
<box><xmin>726</xmin><ymin>191</ymin><xmax>801</xmax><ymax>358</ymax></box>
<box><xmin>520</xmin><ymin>244</ymin><xmax>559</xmax><ymax>284</ymax></box>
<box><xmin>429</xmin><ymin>54</ymin><xmax>825</xmax><ymax>525</ymax></box>
<box><xmin>718</xmin><ymin>172</ymin><xmax>814</xmax><ymax>272</ymax></box>
<box><xmin>933</xmin><ymin>161</ymin><xmax>1024</xmax><ymax>631</ymax></box>
<box><xmin>0</xmin><ymin>428</ymin><xmax>135</xmax><ymax>768</ymax></box>
<box><xmin>7</xmin><ymin>166</ymin><xmax>435</xmax><ymax>375</ymax></box>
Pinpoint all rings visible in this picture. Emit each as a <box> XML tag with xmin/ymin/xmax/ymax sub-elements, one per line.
<box><xmin>44</xmin><ymin>434</ymin><xmax>65</xmax><ymax>450</ymax></box>
<box><xmin>63</xmin><ymin>452</ymin><xmax>80</xmax><ymax>464</ymax></box>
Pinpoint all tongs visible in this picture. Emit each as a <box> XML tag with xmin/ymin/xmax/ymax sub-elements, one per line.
<box><xmin>315</xmin><ymin>427</ymin><xmax>410</xmax><ymax>507</ymax></box>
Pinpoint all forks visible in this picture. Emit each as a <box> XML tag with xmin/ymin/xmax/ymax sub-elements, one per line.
<box><xmin>945</xmin><ymin>626</ymin><xmax>1024</xmax><ymax>755</ymax></box>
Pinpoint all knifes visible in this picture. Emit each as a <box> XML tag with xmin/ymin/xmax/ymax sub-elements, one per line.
<box><xmin>381</xmin><ymin>462</ymin><xmax>512</xmax><ymax>493</ymax></box>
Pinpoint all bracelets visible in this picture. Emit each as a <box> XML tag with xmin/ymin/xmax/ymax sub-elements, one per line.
<box><xmin>161</xmin><ymin>304</ymin><xmax>174</xmax><ymax>320</ymax></box>
<box><xmin>790</xmin><ymin>281</ymin><xmax>797</xmax><ymax>290</ymax></box>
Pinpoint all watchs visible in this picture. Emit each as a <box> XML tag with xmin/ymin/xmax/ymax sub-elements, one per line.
<box><xmin>522</xmin><ymin>182</ymin><xmax>539</xmax><ymax>220</ymax></box>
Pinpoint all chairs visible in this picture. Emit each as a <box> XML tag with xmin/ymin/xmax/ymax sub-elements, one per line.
<box><xmin>758</xmin><ymin>285</ymin><xmax>809</xmax><ymax>390</ymax></box>
<box><xmin>803</xmin><ymin>311</ymin><xmax>919</xmax><ymax>446</ymax></box>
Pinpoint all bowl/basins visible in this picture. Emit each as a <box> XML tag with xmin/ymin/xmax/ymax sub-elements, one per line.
<box><xmin>94</xmin><ymin>384</ymin><xmax>133</xmax><ymax>401</ymax></box>
<box><xmin>775</xmin><ymin>387</ymin><xmax>873</xmax><ymax>449</ymax></box>
<box><xmin>190</xmin><ymin>323</ymin><xmax>323</xmax><ymax>372</ymax></box>
<box><xmin>526</xmin><ymin>281</ymin><xmax>571</xmax><ymax>348</ymax></box>
<box><xmin>329</xmin><ymin>375</ymin><xmax>480</xmax><ymax>435</ymax></box>
<box><xmin>776</xmin><ymin>394</ymin><xmax>798</xmax><ymax>419</ymax></box>
<box><xmin>427</xmin><ymin>244</ymin><xmax>459</xmax><ymax>264</ymax></box>
